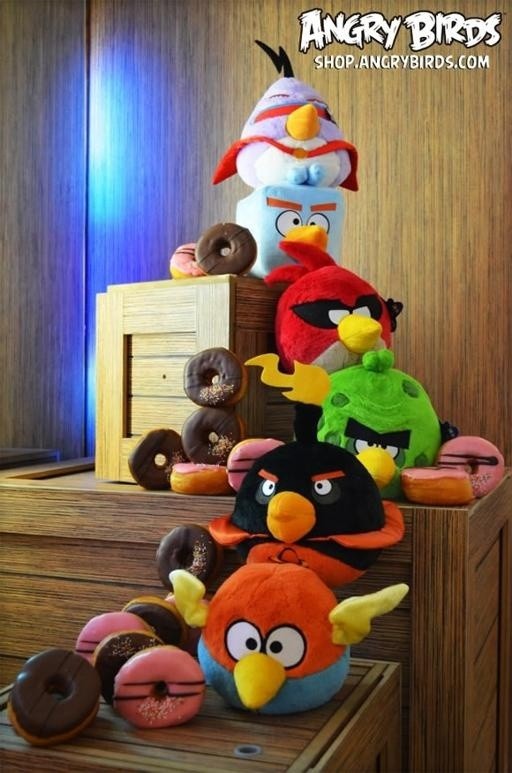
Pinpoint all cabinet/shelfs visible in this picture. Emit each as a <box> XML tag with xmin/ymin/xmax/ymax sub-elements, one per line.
<box><xmin>0</xmin><ymin>456</ymin><xmax>510</xmax><ymax>773</ymax></box>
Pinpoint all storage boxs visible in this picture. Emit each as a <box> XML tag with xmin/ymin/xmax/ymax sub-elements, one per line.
<box><xmin>89</xmin><ymin>274</ymin><xmax>296</xmax><ymax>484</ymax></box>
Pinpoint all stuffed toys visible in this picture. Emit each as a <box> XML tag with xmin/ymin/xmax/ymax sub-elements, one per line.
<box><xmin>167</xmin><ymin>563</ymin><xmax>411</xmax><ymax>719</ymax></box>
<box><xmin>207</xmin><ymin>439</ymin><xmax>404</xmax><ymax>591</ymax></box>
<box><xmin>263</xmin><ymin>238</ymin><xmax>408</xmax><ymax>374</ymax></box>
<box><xmin>208</xmin><ymin>39</ymin><xmax>359</xmax><ymax>192</ymax></box>
<box><xmin>244</xmin><ymin>351</ymin><xmax>458</xmax><ymax>503</ymax></box>
<box><xmin>233</xmin><ymin>183</ymin><xmax>345</xmax><ymax>280</ymax></box>
